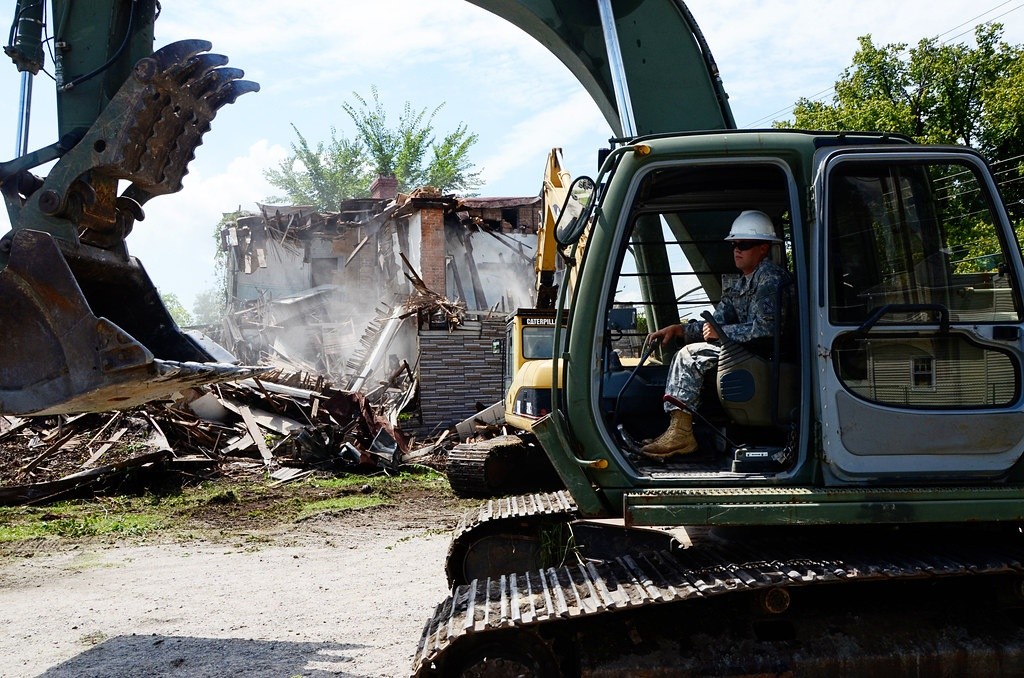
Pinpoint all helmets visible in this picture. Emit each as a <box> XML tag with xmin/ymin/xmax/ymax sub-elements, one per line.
<box><xmin>724</xmin><ymin>210</ymin><xmax>782</xmax><ymax>243</ymax></box>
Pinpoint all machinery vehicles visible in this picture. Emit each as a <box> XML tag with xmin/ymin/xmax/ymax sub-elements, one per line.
<box><xmin>2</xmin><ymin>0</ymin><xmax>1023</xmax><ymax>678</ymax></box>
<box><xmin>444</xmin><ymin>146</ymin><xmax>664</xmax><ymax>502</ymax></box>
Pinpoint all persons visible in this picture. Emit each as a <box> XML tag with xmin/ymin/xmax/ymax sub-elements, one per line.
<box><xmin>642</xmin><ymin>210</ymin><xmax>791</xmax><ymax>457</ymax></box>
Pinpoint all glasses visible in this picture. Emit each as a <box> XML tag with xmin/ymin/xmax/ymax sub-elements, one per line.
<box><xmin>728</xmin><ymin>241</ymin><xmax>765</xmax><ymax>251</ymax></box>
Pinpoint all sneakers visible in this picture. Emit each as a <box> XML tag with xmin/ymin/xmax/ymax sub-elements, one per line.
<box><xmin>641</xmin><ymin>411</ymin><xmax>698</xmax><ymax>457</ymax></box>
